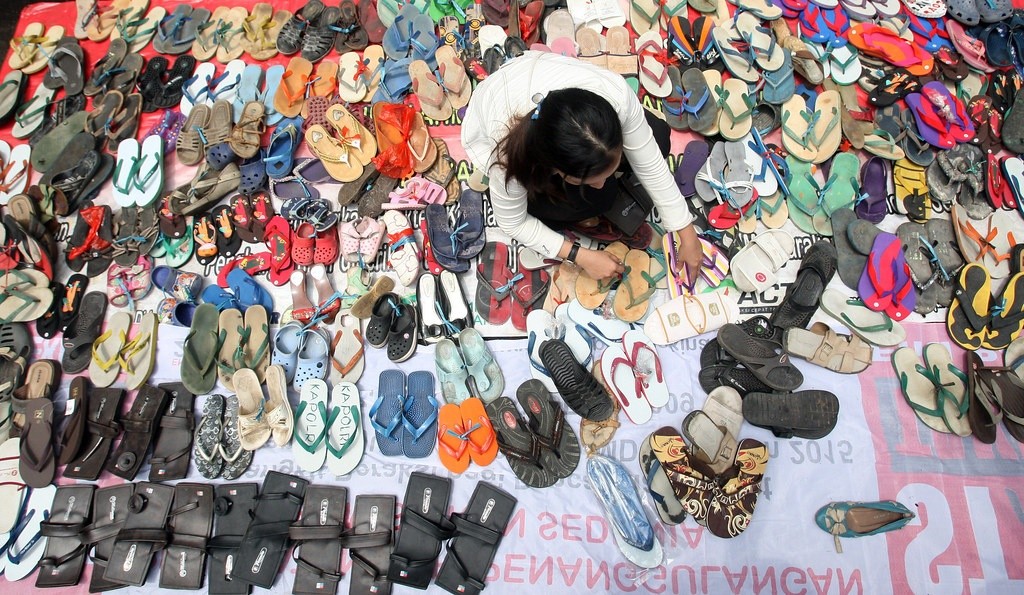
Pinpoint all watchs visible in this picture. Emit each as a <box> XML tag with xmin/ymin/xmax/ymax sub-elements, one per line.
<box><xmin>562</xmin><ymin>242</ymin><xmax>581</xmax><ymax>269</ymax></box>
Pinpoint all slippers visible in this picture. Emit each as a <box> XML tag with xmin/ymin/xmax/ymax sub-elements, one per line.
<box><xmin>0</xmin><ymin>0</ymin><xmax>1024</xmax><ymax>595</ymax></box>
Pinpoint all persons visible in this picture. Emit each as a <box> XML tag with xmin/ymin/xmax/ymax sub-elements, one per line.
<box><xmin>460</xmin><ymin>50</ymin><xmax>703</xmax><ymax>283</ymax></box>
